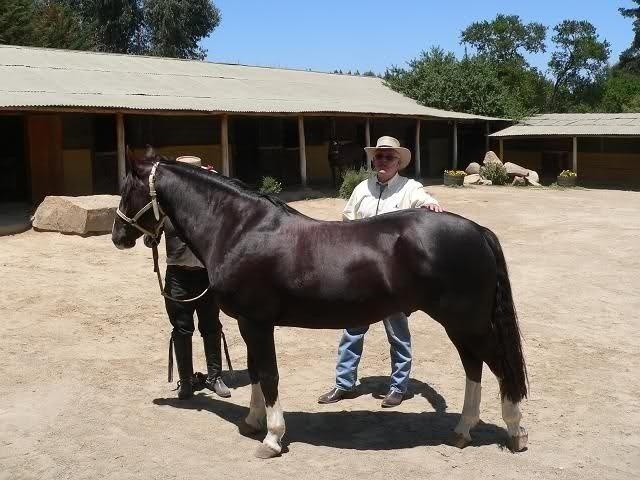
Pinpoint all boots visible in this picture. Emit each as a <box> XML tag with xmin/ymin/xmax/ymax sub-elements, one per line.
<box><xmin>204</xmin><ymin>333</ymin><xmax>232</xmax><ymax>398</ymax></box>
<box><xmin>171</xmin><ymin>335</ymin><xmax>194</xmax><ymax>400</ymax></box>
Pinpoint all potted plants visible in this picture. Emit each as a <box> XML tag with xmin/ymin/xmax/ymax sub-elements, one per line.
<box><xmin>444</xmin><ymin>168</ymin><xmax>468</xmax><ymax>186</ymax></box>
<box><xmin>556</xmin><ymin>169</ymin><xmax>578</xmax><ymax>187</ymax></box>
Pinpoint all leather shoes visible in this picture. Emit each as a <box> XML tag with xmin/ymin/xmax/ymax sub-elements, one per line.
<box><xmin>318</xmin><ymin>388</ymin><xmax>356</xmax><ymax>405</ymax></box>
<box><xmin>382</xmin><ymin>389</ymin><xmax>403</xmax><ymax>408</ymax></box>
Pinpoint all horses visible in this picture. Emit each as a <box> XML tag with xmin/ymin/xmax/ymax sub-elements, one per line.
<box><xmin>111</xmin><ymin>145</ymin><xmax>530</xmax><ymax>459</ymax></box>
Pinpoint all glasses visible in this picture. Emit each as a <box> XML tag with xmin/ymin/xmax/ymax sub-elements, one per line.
<box><xmin>373</xmin><ymin>153</ymin><xmax>400</xmax><ymax>161</ymax></box>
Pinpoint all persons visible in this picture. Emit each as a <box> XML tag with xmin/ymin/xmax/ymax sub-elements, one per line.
<box><xmin>315</xmin><ymin>135</ymin><xmax>447</xmax><ymax>410</ymax></box>
<box><xmin>142</xmin><ymin>154</ymin><xmax>232</xmax><ymax>399</ymax></box>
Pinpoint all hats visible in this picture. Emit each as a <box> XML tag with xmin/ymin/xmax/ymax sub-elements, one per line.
<box><xmin>176</xmin><ymin>155</ymin><xmax>217</xmax><ymax>176</ymax></box>
<box><xmin>363</xmin><ymin>135</ymin><xmax>411</xmax><ymax>171</ymax></box>
